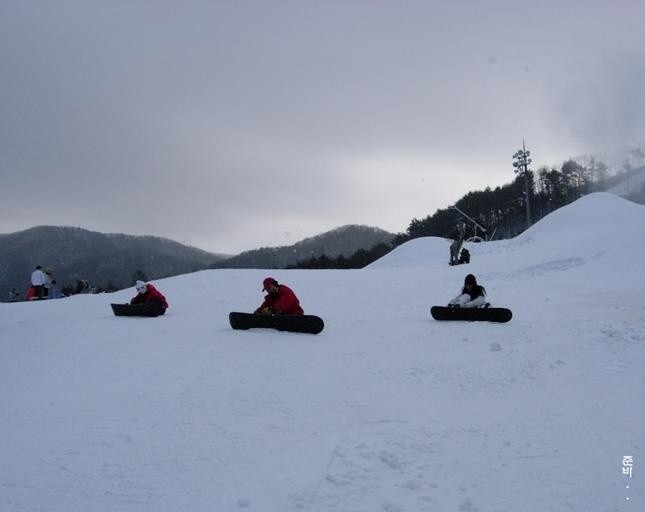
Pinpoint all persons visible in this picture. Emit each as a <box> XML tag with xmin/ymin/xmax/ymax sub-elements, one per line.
<box><xmin>25</xmin><ymin>284</ymin><xmax>35</xmax><ymax>300</ymax></box>
<box><xmin>30</xmin><ymin>265</ymin><xmax>46</xmax><ymax>300</ymax></box>
<box><xmin>42</xmin><ymin>268</ymin><xmax>53</xmax><ymax>299</ymax></box>
<box><xmin>254</xmin><ymin>276</ymin><xmax>305</xmax><ymax>315</ymax></box>
<box><xmin>48</xmin><ymin>281</ymin><xmax>61</xmax><ymax>299</ymax></box>
<box><xmin>448</xmin><ymin>236</ymin><xmax>462</xmax><ymax>265</ymax></box>
<box><xmin>453</xmin><ymin>248</ymin><xmax>471</xmax><ymax>264</ymax></box>
<box><xmin>447</xmin><ymin>272</ymin><xmax>487</xmax><ymax>308</ymax></box>
<box><xmin>131</xmin><ymin>280</ymin><xmax>168</xmax><ymax>316</ymax></box>
<box><xmin>456</xmin><ymin>216</ymin><xmax>466</xmax><ymax>243</ymax></box>
<box><xmin>10</xmin><ymin>287</ymin><xmax>20</xmax><ymax>302</ymax></box>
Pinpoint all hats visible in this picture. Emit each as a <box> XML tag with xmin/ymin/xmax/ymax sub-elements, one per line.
<box><xmin>464</xmin><ymin>274</ymin><xmax>476</xmax><ymax>285</ymax></box>
<box><xmin>262</xmin><ymin>277</ymin><xmax>275</xmax><ymax>292</ymax></box>
<box><xmin>36</xmin><ymin>265</ymin><xmax>43</xmax><ymax>270</ymax></box>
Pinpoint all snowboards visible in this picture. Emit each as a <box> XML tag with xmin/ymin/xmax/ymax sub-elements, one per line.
<box><xmin>229</xmin><ymin>312</ymin><xmax>324</xmax><ymax>334</ymax></box>
<box><xmin>430</xmin><ymin>306</ymin><xmax>512</xmax><ymax>323</ymax></box>
<box><xmin>110</xmin><ymin>303</ymin><xmax>162</xmax><ymax>318</ymax></box>
